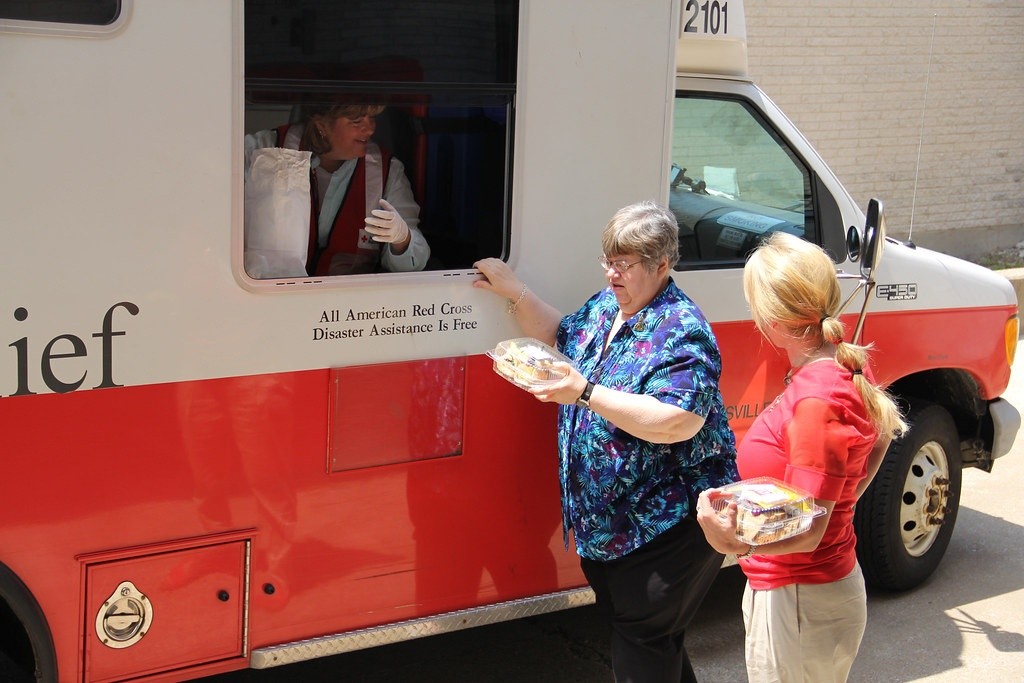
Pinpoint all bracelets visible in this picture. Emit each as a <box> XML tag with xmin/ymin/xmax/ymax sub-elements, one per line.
<box><xmin>736</xmin><ymin>544</ymin><xmax>756</xmax><ymax>560</ymax></box>
<box><xmin>508</xmin><ymin>283</ymin><xmax>528</xmax><ymax>314</ymax></box>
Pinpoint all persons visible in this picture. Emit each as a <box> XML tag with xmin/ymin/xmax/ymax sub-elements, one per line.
<box><xmin>242</xmin><ymin>54</ymin><xmax>432</xmax><ymax>277</ymax></box>
<box><xmin>470</xmin><ymin>200</ymin><xmax>744</xmax><ymax>683</ymax></box>
<box><xmin>696</xmin><ymin>230</ymin><xmax>910</xmax><ymax>683</ymax></box>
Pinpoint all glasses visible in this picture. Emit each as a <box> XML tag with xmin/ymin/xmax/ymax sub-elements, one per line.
<box><xmin>598</xmin><ymin>254</ymin><xmax>644</xmax><ymax>273</ymax></box>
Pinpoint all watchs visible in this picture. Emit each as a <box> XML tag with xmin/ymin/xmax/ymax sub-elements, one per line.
<box><xmin>575</xmin><ymin>380</ymin><xmax>597</xmax><ymax>409</ymax></box>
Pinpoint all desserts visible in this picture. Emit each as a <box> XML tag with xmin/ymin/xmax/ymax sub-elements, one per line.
<box><xmin>719</xmin><ymin>503</ymin><xmax>802</xmax><ymax>545</ymax></box>
<box><xmin>497</xmin><ymin>342</ymin><xmax>567</xmax><ymax>388</ymax></box>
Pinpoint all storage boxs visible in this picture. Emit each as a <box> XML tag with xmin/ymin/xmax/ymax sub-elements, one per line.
<box><xmin>485</xmin><ymin>337</ymin><xmax>577</xmax><ymax>391</ymax></box>
<box><xmin>711</xmin><ymin>476</ymin><xmax>827</xmax><ymax>545</ymax></box>
